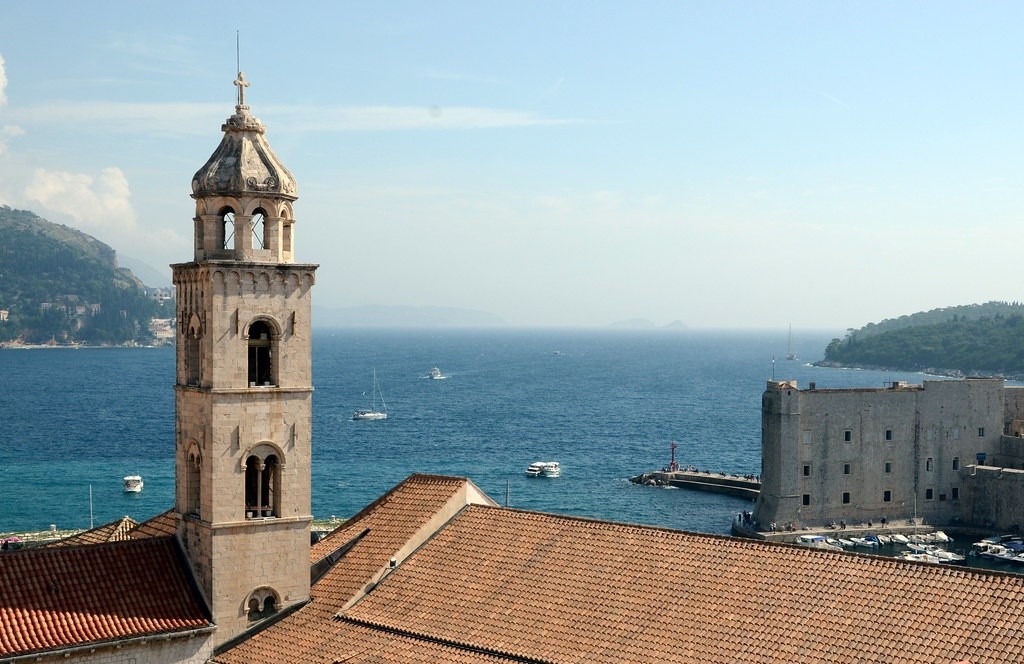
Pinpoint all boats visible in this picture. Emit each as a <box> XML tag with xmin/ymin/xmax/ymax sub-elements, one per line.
<box><xmin>429</xmin><ymin>366</ymin><xmax>442</xmax><ymax>379</ymax></box>
<box><xmin>876</xmin><ymin>534</ymin><xmax>890</xmax><ymax>544</ymax></box>
<box><xmin>526</xmin><ymin>462</ymin><xmax>544</xmax><ymax>478</ymax></box>
<box><xmin>543</xmin><ymin>461</ymin><xmax>561</xmax><ymax>478</ymax></box>
<box><xmin>825</xmin><ymin>535</ymin><xmax>842</xmax><ymax>550</ymax></box>
<box><xmin>848</xmin><ymin>535</ymin><xmax>878</xmax><ymax>548</ymax></box>
<box><xmin>972</xmin><ymin>533</ymin><xmax>1024</xmax><ymax>564</ymax></box>
<box><xmin>897</xmin><ymin>529</ymin><xmax>965</xmax><ymax>563</ymax></box>
<box><xmin>121</xmin><ymin>474</ymin><xmax>144</xmax><ymax>492</ymax></box>
<box><xmin>837</xmin><ymin>537</ymin><xmax>854</xmax><ymax>547</ymax></box>
<box><xmin>892</xmin><ymin>532</ymin><xmax>909</xmax><ymax>543</ymax></box>
<box><xmin>796</xmin><ymin>534</ymin><xmax>842</xmax><ymax>550</ymax></box>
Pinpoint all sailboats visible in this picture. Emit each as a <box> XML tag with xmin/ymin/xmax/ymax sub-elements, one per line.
<box><xmin>353</xmin><ymin>367</ymin><xmax>388</xmax><ymax>421</ymax></box>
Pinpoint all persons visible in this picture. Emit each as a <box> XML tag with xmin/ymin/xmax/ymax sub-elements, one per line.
<box><xmin>737</xmin><ymin>510</ymin><xmax>759</xmax><ymax>530</ymax></box>
<box><xmin>840</xmin><ymin>519</ymin><xmax>847</xmax><ymax>529</ymax></box>
<box><xmin>868</xmin><ymin>518</ymin><xmax>872</xmax><ymax>527</ymax></box>
<box><xmin>828</xmin><ymin>520</ymin><xmax>835</xmax><ymax>527</ymax></box>
<box><xmin>770</xmin><ymin>522</ymin><xmax>776</xmax><ymax>533</ymax></box>
<box><xmin>881</xmin><ymin>516</ymin><xmax>886</xmax><ymax>528</ymax></box>
<box><xmin>786</xmin><ymin>521</ymin><xmax>810</xmax><ymax>531</ymax></box>
